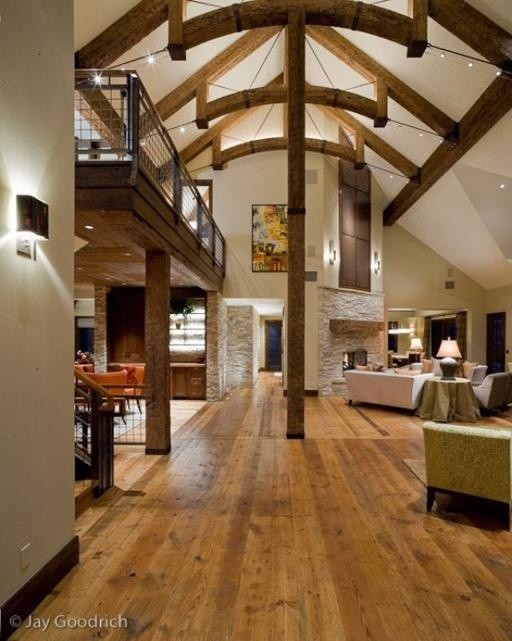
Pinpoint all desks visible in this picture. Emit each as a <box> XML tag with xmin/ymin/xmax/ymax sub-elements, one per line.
<box><xmin>415</xmin><ymin>376</ymin><xmax>481</xmax><ymax>424</ymax></box>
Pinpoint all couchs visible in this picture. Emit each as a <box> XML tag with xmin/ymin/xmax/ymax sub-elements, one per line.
<box><xmin>473</xmin><ymin>373</ymin><xmax>512</xmax><ymax>416</ymax></box>
<box><xmin>410</xmin><ymin>358</ymin><xmax>488</xmax><ymax>387</ymax></box>
<box><xmin>423</xmin><ymin>356</ymin><xmax>479</xmax><ymax>378</ymax></box>
<box><xmin>344</xmin><ymin>369</ymin><xmax>435</xmax><ymax>417</ymax></box>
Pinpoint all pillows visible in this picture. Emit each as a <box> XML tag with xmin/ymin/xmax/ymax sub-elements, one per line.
<box><xmin>355</xmin><ymin>363</ymin><xmax>423</xmax><ymax>375</ymax></box>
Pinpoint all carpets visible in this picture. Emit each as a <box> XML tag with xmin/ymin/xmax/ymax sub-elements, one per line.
<box><xmin>74</xmin><ymin>399</ymin><xmax>146</xmax><ymax>439</ymax></box>
<box><xmin>402</xmin><ymin>456</ymin><xmax>467</xmax><ymax>513</ymax></box>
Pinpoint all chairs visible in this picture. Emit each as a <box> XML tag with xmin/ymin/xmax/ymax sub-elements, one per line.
<box><xmin>422</xmin><ymin>420</ymin><xmax>512</xmax><ymax>531</ymax></box>
<box><xmin>67</xmin><ymin>363</ymin><xmax>146</xmax><ymax>426</ymax></box>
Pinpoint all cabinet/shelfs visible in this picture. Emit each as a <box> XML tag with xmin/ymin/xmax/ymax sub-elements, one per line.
<box><xmin>171</xmin><ymin>367</ymin><xmax>207</xmax><ymax>400</ymax></box>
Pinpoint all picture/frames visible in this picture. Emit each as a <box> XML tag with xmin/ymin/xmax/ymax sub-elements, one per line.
<box><xmin>251</xmin><ymin>203</ymin><xmax>288</xmax><ymax>272</ymax></box>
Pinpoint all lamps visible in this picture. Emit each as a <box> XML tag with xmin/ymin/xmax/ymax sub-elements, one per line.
<box><xmin>435</xmin><ymin>337</ymin><xmax>462</xmax><ymax>381</ymax></box>
<box><xmin>14</xmin><ymin>194</ymin><xmax>49</xmax><ymax>243</ymax></box>
<box><xmin>387</xmin><ymin>320</ymin><xmax>415</xmax><ymax>336</ymax></box>
<box><xmin>408</xmin><ymin>338</ymin><xmax>425</xmax><ymax>362</ymax></box>
